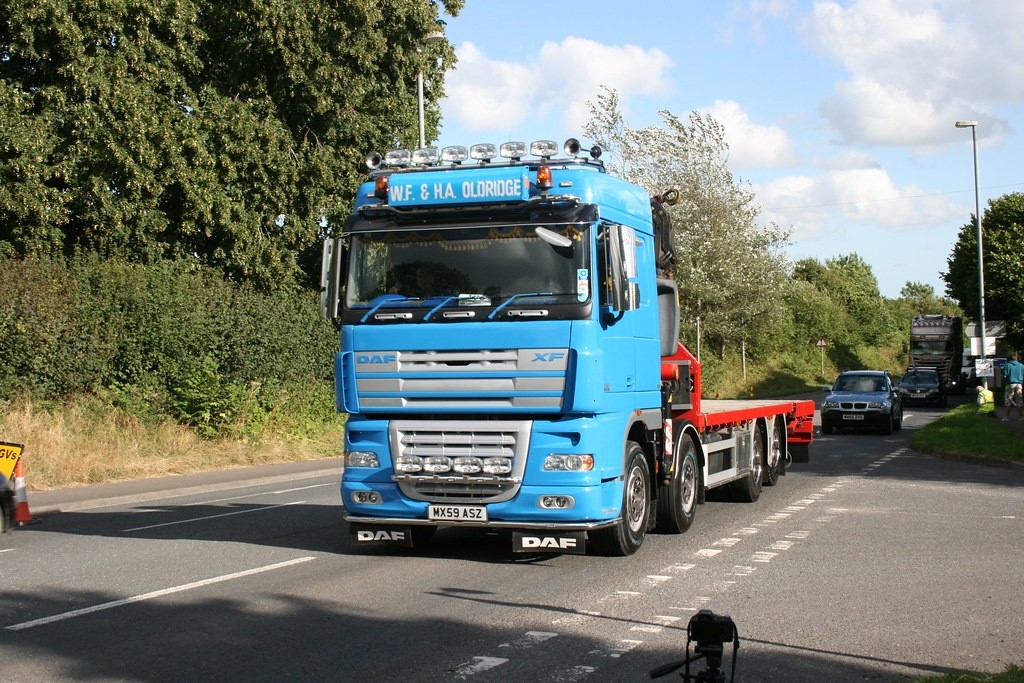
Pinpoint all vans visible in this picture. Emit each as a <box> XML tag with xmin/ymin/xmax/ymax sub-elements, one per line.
<box><xmin>962</xmin><ymin>348</ymin><xmax>980</xmax><ymax>380</ymax></box>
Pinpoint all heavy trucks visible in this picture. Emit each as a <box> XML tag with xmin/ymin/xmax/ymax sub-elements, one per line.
<box><xmin>901</xmin><ymin>315</ymin><xmax>969</xmax><ymax>397</ymax></box>
<box><xmin>319</xmin><ymin>139</ymin><xmax>817</xmax><ymax>557</ymax></box>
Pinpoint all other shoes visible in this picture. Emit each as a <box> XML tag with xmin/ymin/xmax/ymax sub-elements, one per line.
<box><xmin>1001</xmin><ymin>417</ymin><xmax>1010</xmax><ymax>423</ymax></box>
<box><xmin>1016</xmin><ymin>417</ymin><xmax>1024</xmax><ymax>423</ymax></box>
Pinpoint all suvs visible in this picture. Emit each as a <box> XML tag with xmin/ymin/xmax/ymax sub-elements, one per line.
<box><xmin>820</xmin><ymin>370</ymin><xmax>903</xmax><ymax>433</ymax></box>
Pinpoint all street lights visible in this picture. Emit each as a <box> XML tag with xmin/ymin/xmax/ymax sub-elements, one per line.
<box><xmin>415</xmin><ymin>30</ymin><xmax>445</xmax><ymax>148</ymax></box>
<box><xmin>956</xmin><ymin>118</ymin><xmax>989</xmax><ymax>394</ymax></box>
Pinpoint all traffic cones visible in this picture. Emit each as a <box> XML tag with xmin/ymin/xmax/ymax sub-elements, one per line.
<box><xmin>13</xmin><ymin>460</ymin><xmax>35</xmax><ymax>527</ymax></box>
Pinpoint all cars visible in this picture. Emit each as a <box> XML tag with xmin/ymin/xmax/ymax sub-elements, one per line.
<box><xmin>898</xmin><ymin>370</ymin><xmax>945</xmax><ymax>409</ymax></box>
<box><xmin>993</xmin><ymin>358</ymin><xmax>1007</xmax><ymax>370</ymax></box>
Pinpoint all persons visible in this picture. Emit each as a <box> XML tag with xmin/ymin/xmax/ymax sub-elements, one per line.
<box><xmin>1002</xmin><ymin>353</ymin><xmax>1024</xmax><ymax>422</ymax></box>
<box><xmin>976</xmin><ymin>385</ymin><xmax>999</xmax><ymax>419</ymax></box>
